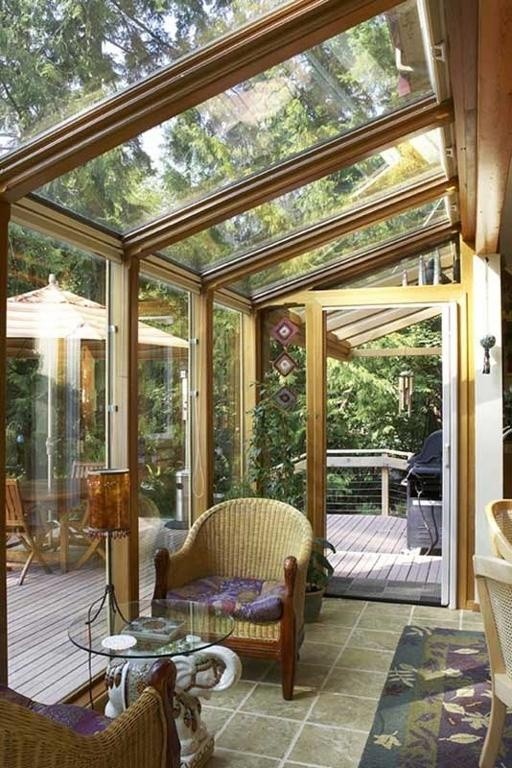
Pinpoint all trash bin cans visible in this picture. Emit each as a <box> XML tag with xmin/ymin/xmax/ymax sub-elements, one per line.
<box><xmin>164</xmin><ymin>471</ymin><xmax>192</xmax><ymax>531</ymax></box>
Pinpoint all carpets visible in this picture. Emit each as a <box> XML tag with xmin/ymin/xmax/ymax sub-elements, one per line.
<box><xmin>356</xmin><ymin>626</ymin><xmax>511</xmax><ymax>768</ymax></box>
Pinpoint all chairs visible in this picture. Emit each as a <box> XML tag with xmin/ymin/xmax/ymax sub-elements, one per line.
<box><xmin>1</xmin><ymin>659</ymin><xmax>180</xmax><ymax>768</ymax></box>
<box><xmin>472</xmin><ymin>499</ymin><xmax>512</xmax><ymax>768</ymax></box>
<box><xmin>152</xmin><ymin>499</ymin><xmax>313</xmax><ymax>700</ymax></box>
<box><xmin>5</xmin><ymin>461</ymin><xmax>106</xmax><ymax>586</ymax></box>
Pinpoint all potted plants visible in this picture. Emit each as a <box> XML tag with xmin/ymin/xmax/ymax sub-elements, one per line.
<box><xmin>305</xmin><ymin>538</ymin><xmax>335</xmax><ymax>622</ymax></box>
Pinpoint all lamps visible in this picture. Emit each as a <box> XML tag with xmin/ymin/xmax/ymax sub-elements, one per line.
<box><xmin>86</xmin><ymin>469</ymin><xmax>131</xmax><ymax>627</ymax></box>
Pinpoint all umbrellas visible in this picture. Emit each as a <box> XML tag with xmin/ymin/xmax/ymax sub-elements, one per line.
<box><xmin>7</xmin><ymin>273</ymin><xmax>189</xmax><ymax>547</ymax></box>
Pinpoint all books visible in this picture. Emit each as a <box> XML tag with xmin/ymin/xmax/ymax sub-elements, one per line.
<box><xmin>121</xmin><ymin>616</ymin><xmax>186</xmax><ymax>641</ymax></box>
